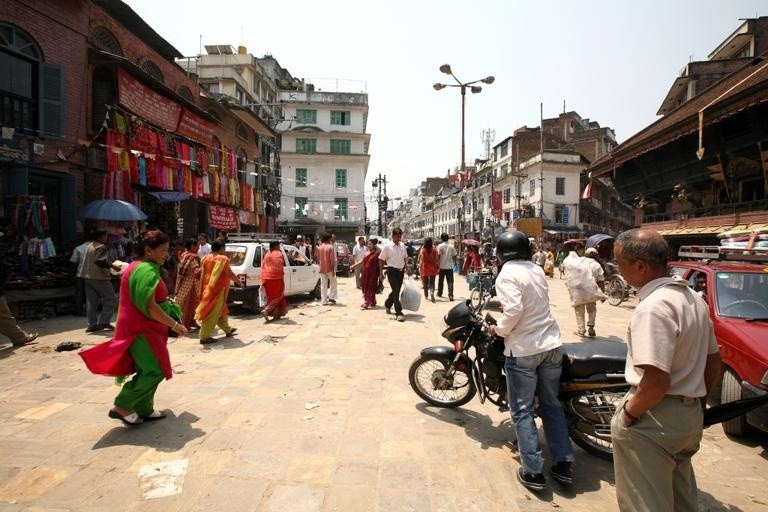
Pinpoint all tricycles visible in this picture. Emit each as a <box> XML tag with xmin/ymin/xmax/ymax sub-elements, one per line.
<box><xmin>466</xmin><ymin>270</ymin><xmax>499</xmax><ymax>310</ymax></box>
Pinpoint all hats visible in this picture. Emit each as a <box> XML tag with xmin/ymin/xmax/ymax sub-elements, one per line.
<box><xmin>584</xmin><ymin>247</ymin><xmax>598</xmax><ymax>254</ymax></box>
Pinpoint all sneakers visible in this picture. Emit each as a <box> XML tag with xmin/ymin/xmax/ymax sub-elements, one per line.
<box><xmin>200</xmin><ymin>337</ymin><xmax>218</xmax><ymax>344</ymax></box>
<box><xmin>261</xmin><ymin>310</ymin><xmax>270</xmax><ymax>321</ymax></box>
<box><xmin>517</xmin><ymin>466</ymin><xmax>546</xmax><ymax>491</ymax></box>
<box><xmin>227</xmin><ymin>328</ymin><xmax>237</xmax><ymax>336</ymax></box>
<box><xmin>550</xmin><ymin>462</ymin><xmax>574</xmax><ymax>483</ymax></box>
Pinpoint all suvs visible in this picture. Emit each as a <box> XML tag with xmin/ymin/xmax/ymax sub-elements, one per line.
<box><xmin>200</xmin><ymin>232</ymin><xmax>330</xmax><ymax>314</ymax></box>
<box><xmin>314</xmin><ymin>242</ymin><xmax>355</xmax><ymax>277</ymax></box>
<box><xmin>666</xmin><ymin>245</ymin><xmax>767</xmax><ymax>437</ymax></box>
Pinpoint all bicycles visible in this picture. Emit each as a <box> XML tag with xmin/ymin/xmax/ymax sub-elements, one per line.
<box><xmin>602</xmin><ymin>273</ymin><xmax>628</xmax><ymax>305</ymax></box>
<box><xmin>555</xmin><ymin>259</ymin><xmax>564</xmax><ymax>278</ymax></box>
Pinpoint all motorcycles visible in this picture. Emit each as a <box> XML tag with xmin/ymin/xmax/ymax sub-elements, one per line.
<box><xmin>409</xmin><ymin>268</ymin><xmax>631</xmax><ymax>463</ymax></box>
<box><xmin>404</xmin><ymin>253</ymin><xmax>417</xmax><ymax>276</ymax></box>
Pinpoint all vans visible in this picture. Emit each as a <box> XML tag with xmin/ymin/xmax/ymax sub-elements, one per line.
<box><xmin>353</xmin><ymin>235</ymin><xmax>388</xmax><ymax>250</ymax></box>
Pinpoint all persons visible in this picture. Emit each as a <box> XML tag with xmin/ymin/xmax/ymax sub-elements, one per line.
<box><xmin>68</xmin><ymin>229</ymin><xmax>497</xmax><ymax>345</ymax></box>
<box><xmin>558</xmin><ymin>238</ymin><xmax>608</xmax><ymax>338</ymax></box>
<box><xmin>607</xmin><ymin>226</ymin><xmax>724</xmax><ymax>511</ymax></box>
<box><xmin>694</xmin><ymin>271</ymin><xmax>743</xmax><ymax>311</ymax></box>
<box><xmin>488</xmin><ymin>230</ymin><xmax>574</xmax><ymax>493</ymax></box>
<box><xmin>80</xmin><ymin>230</ymin><xmax>191</xmax><ymax>426</ymax></box>
<box><xmin>1</xmin><ymin>291</ymin><xmax>39</xmax><ymax>347</ymax></box>
<box><xmin>529</xmin><ymin>238</ymin><xmax>606</xmax><ymax>280</ymax></box>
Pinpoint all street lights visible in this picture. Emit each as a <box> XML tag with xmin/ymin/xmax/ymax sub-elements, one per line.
<box><xmin>433</xmin><ymin>64</ymin><xmax>495</xmax><ymax>276</ymax></box>
<box><xmin>378</xmin><ymin>197</ymin><xmax>402</xmax><ymax>237</ymax></box>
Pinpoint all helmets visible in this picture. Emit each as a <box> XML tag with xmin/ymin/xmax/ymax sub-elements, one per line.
<box><xmin>496</xmin><ymin>231</ymin><xmax>532</xmax><ymax>264</ymax></box>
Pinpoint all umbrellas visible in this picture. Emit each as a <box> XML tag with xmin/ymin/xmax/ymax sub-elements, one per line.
<box><xmin>79</xmin><ymin>196</ymin><xmax>148</xmax><ymax>224</ymax></box>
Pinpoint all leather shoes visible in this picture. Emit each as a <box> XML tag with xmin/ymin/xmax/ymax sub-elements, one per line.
<box><xmin>329</xmin><ymin>299</ymin><xmax>336</xmax><ymax>304</ymax></box>
<box><xmin>25</xmin><ymin>332</ymin><xmax>39</xmax><ymax>342</ymax></box>
<box><xmin>88</xmin><ymin>323</ymin><xmax>114</xmax><ymax>331</ymax></box>
<box><xmin>385</xmin><ymin>303</ymin><xmax>405</xmax><ymax>320</ymax></box>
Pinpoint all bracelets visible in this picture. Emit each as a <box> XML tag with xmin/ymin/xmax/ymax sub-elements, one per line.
<box><xmin>622</xmin><ymin>399</ymin><xmax>640</xmax><ymax>423</ymax></box>
<box><xmin>171</xmin><ymin>321</ymin><xmax>178</xmax><ymax>332</ymax></box>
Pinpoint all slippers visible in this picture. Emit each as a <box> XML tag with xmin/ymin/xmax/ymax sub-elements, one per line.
<box><xmin>109</xmin><ymin>410</ymin><xmax>144</xmax><ymax>425</ymax></box>
<box><xmin>141</xmin><ymin>410</ymin><xmax>166</xmax><ymax>420</ymax></box>
